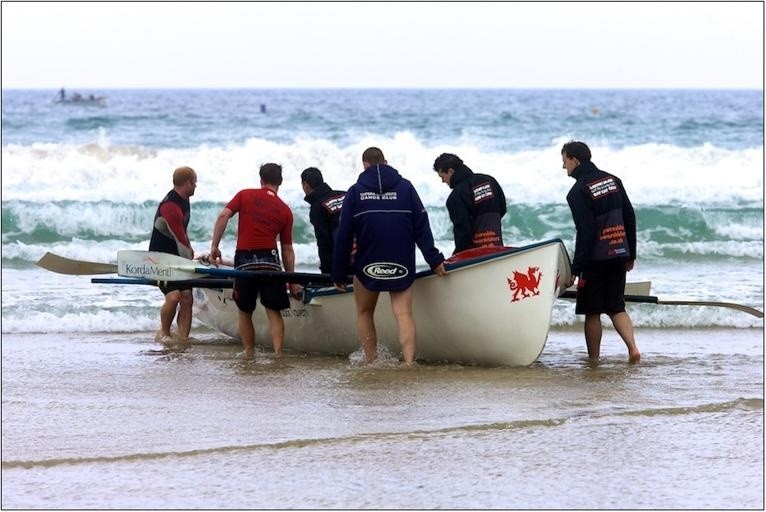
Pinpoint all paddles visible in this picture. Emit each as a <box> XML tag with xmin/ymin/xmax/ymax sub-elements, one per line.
<box><xmin>117</xmin><ymin>249</ymin><xmax>353</xmax><ymax>285</ymax></box>
<box><xmin>443</xmin><ymin>245</ymin><xmax>518</xmax><ymax>262</ymax></box>
<box><xmin>37</xmin><ymin>252</ymin><xmax>118</xmax><ymax>275</ymax></box>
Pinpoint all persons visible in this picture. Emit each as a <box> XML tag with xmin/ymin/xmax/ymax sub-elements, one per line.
<box><xmin>434</xmin><ymin>153</ymin><xmax>508</xmax><ymax>256</ymax></box>
<box><xmin>147</xmin><ymin>167</ymin><xmax>199</xmax><ymax>337</ymax></box>
<box><xmin>560</xmin><ymin>140</ymin><xmax>641</xmax><ymax>364</ymax></box>
<box><xmin>210</xmin><ymin>162</ymin><xmax>301</xmax><ymax>354</ymax></box>
<box><xmin>332</xmin><ymin>147</ymin><xmax>448</xmax><ymax>363</ymax></box>
<box><xmin>300</xmin><ymin>167</ymin><xmax>357</xmax><ymax>287</ymax></box>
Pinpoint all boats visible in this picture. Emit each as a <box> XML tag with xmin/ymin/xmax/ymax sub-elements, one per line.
<box><xmin>192</xmin><ymin>238</ymin><xmax>573</xmax><ymax>368</ymax></box>
<box><xmin>53</xmin><ymin>96</ymin><xmax>106</xmax><ymax>107</ymax></box>
